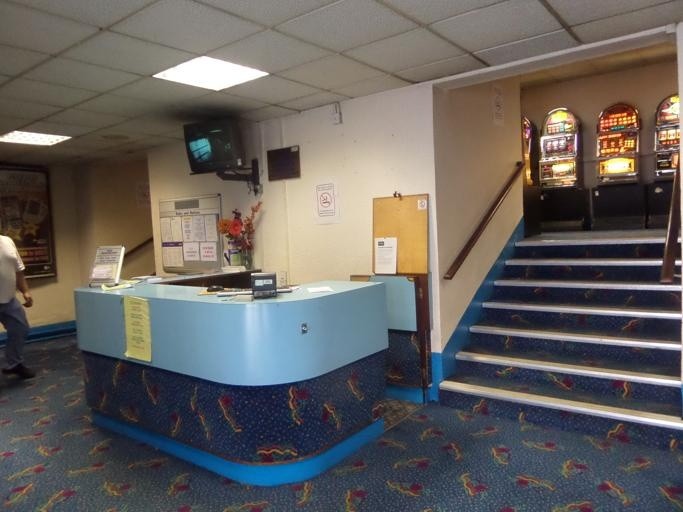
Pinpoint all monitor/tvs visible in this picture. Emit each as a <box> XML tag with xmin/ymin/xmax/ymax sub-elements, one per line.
<box><xmin>183</xmin><ymin>118</ymin><xmax>246</xmax><ymax>175</ymax></box>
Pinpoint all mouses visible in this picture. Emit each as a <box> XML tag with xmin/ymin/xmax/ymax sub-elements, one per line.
<box><xmin>207</xmin><ymin>285</ymin><xmax>224</xmax><ymax>292</ymax></box>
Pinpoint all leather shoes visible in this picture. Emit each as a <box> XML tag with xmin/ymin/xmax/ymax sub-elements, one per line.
<box><xmin>3</xmin><ymin>363</ymin><xmax>34</xmax><ymax>377</ymax></box>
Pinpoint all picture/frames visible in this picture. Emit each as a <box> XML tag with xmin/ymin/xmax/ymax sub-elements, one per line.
<box><xmin>1</xmin><ymin>167</ymin><xmax>56</xmax><ymax>281</ymax></box>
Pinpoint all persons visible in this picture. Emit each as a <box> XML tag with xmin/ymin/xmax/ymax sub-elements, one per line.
<box><xmin>0</xmin><ymin>210</ymin><xmax>35</xmax><ymax>378</ymax></box>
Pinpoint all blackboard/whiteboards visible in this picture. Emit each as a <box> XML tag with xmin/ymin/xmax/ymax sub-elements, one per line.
<box><xmin>158</xmin><ymin>192</ymin><xmax>222</xmax><ymax>274</ymax></box>
